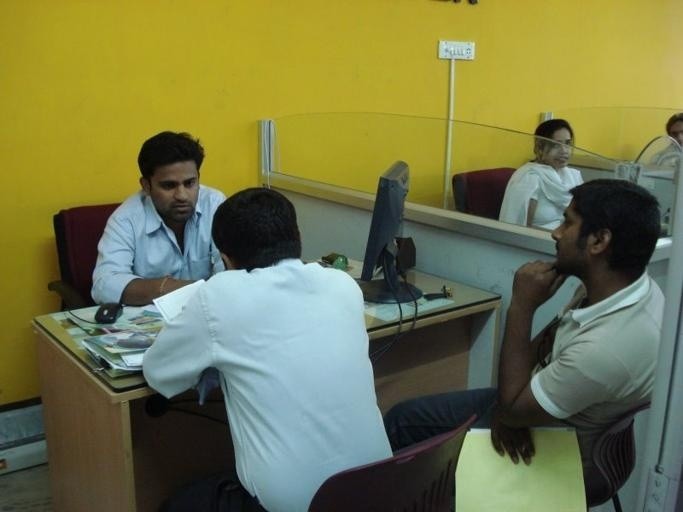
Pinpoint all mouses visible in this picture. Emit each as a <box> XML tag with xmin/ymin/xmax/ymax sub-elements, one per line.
<box><xmin>94</xmin><ymin>302</ymin><xmax>124</xmax><ymax>323</ymax></box>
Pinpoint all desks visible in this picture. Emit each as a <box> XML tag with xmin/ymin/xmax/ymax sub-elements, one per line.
<box><xmin>30</xmin><ymin>258</ymin><xmax>504</xmax><ymax>512</ymax></box>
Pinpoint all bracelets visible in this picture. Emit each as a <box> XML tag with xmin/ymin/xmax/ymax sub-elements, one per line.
<box><xmin>158</xmin><ymin>274</ymin><xmax>171</xmax><ymax>297</ymax></box>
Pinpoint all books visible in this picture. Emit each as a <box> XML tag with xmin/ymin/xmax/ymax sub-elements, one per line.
<box><xmin>82</xmin><ymin>329</ymin><xmax>154</xmax><ymax>379</ymax></box>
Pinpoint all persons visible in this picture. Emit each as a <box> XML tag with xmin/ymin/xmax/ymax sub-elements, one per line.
<box><xmin>89</xmin><ymin>130</ymin><xmax>226</xmax><ymax>307</ymax></box>
<box><xmin>379</xmin><ymin>178</ymin><xmax>664</xmax><ymax>466</ymax></box>
<box><xmin>498</xmin><ymin>120</ymin><xmax>585</xmax><ymax>227</ymax></box>
<box><xmin>141</xmin><ymin>186</ymin><xmax>392</xmax><ymax>512</ymax></box>
<box><xmin>654</xmin><ymin>113</ymin><xmax>682</xmax><ymax>168</ymax></box>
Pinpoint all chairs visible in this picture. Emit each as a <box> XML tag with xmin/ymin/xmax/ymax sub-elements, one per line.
<box><xmin>578</xmin><ymin>402</ymin><xmax>651</xmax><ymax>512</ymax></box>
<box><xmin>46</xmin><ymin>202</ymin><xmax>124</xmax><ymax>311</ymax></box>
<box><xmin>216</xmin><ymin>415</ymin><xmax>478</xmax><ymax>512</ymax></box>
<box><xmin>452</xmin><ymin>168</ymin><xmax>518</xmax><ymax>217</ymax></box>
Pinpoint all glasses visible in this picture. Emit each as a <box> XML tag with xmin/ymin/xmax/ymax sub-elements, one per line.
<box><xmin>536</xmin><ymin>316</ymin><xmax>561</xmax><ymax>368</ymax></box>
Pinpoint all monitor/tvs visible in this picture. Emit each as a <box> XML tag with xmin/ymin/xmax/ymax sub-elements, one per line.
<box><xmin>350</xmin><ymin>160</ymin><xmax>423</xmax><ymax>305</ymax></box>
